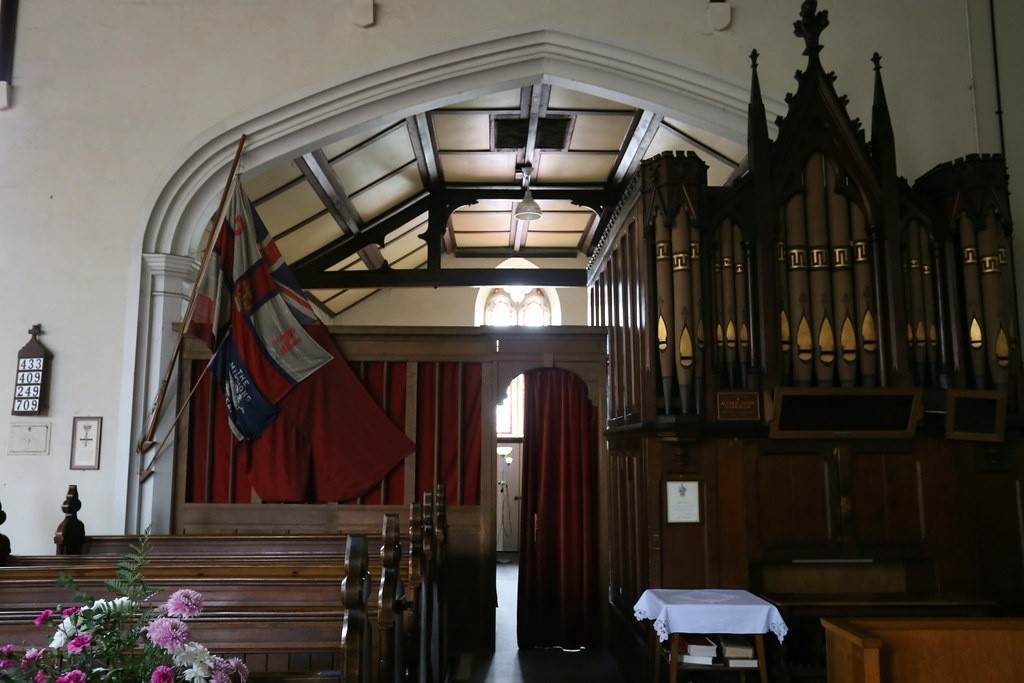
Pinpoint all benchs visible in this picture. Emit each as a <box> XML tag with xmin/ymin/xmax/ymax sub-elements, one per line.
<box><xmin>0</xmin><ymin>482</ymin><xmax>456</xmax><ymax>683</ymax></box>
<box><xmin>818</xmin><ymin>615</ymin><xmax>1024</xmax><ymax>683</ymax></box>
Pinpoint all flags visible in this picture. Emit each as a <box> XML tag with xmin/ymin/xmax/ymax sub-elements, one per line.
<box><xmin>183</xmin><ymin>196</ymin><xmax>416</xmax><ymax>502</ymax></box>
<box><xmin>195</xmin><ymin>174</ymin><xmax>335</xmax><ymax>442</ymax></box>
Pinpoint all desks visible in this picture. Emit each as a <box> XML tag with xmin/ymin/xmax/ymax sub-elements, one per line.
<box><xmin>633</xmin><ymin>588</ymin><xmax>788</xmax><ymax>683</ymax></box>
<box><xmin>758</xmin><ymin>590</ymin><xmax>998</xmax><ymax>683</ymax></box>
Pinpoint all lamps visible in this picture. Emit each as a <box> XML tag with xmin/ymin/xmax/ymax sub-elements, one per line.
<box><xmin>513</xmin><ymin>167</ymin><xmax>544</xmax><ymax>221</ymax></box>
<box><xmin>496</xmin><ymin>446</ymin><xmax>513</xmax><ymax>565</ymax></box>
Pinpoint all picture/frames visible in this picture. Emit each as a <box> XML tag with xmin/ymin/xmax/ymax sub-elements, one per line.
<box><xmin>660</xmin><ymin>472</ymin><xmax>704</xmax><ymax>528</ymax></box>
<box><xmin>69</xmin><ymin>417</ymin><xmax>103</xmax><ymax>470</ymax></box>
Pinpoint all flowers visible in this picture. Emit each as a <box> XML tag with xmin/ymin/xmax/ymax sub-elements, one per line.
<box><xmin>0</xmin><ymin>519</ymin><xmax>250</xmax><ymax>683</ymax></box>
